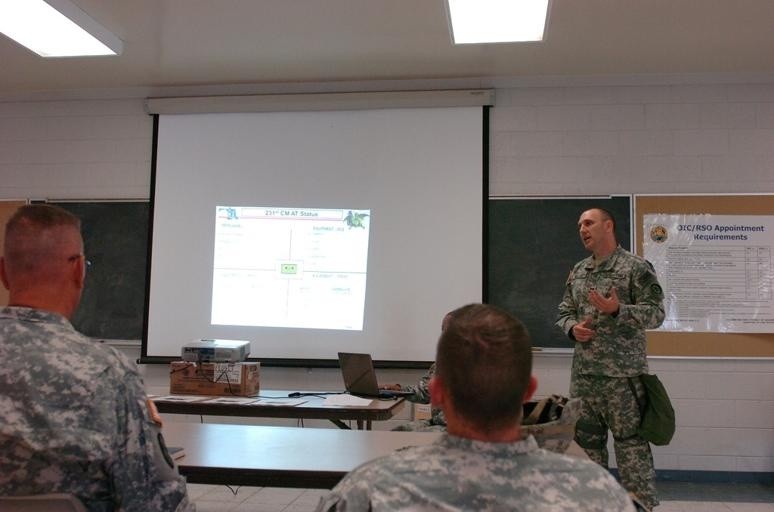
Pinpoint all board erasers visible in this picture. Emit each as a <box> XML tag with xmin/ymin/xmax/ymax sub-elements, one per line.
<box><xmin>531</xmin><ymin>346</ymin><xmax>543</xmax><ymax>351</ymax></box>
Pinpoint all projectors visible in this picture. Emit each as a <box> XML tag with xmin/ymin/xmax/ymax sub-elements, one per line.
<box><xmin>181</xmin><ymin>337</ymin><xmax>252</xmax><ymax>363</ymax></box>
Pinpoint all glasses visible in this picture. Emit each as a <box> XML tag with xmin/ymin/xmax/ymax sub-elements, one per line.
<box><xmin>69</xmin><ymin>252</ymin><xmax>92</xmax><ymax>270</ymax></box>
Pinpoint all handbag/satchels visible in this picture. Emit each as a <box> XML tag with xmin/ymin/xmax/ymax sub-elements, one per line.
<box><xmin>636</xmin><ymin>372</ymin><xmax>676</xmax><ymax>446</ymax></box>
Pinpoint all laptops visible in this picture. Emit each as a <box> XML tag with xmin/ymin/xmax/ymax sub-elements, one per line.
<box><xmin>338</xmin><ymin>352</ymin><xmax>415</xmax><ymax>399</ymax></box>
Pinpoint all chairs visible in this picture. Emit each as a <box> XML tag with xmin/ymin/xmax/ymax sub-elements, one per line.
<box><xmin>1</xmin><ymin>491</ymin><xmax>91</xmax><ymax>511</ymax></box>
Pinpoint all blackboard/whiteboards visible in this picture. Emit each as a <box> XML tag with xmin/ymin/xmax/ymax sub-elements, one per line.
<box><xmin>28</xmin><ymin>194</ymin><xmax>633</xmax><ymax>355</ymax></box>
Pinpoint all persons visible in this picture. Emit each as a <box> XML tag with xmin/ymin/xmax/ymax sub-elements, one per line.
<box><xmin>374</xmin><ymin>308</ymin><xmax>454</xmax><ymax>436</ymax></box>
<box><xmin>551</xmin><ymin>205</ymin><xmax>667</xmax><ymax>512</ymax></box>
<box><xmin>312</xmin><ymin>302</ymin><xmax>639</xmax><ymax>512</ymax></box>
<box><xmin>0</xmin><ymin>202</ymin><xmax>197</xmax><ymax>511</ymax></box>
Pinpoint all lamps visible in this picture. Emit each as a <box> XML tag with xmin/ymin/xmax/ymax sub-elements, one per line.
<box><xmin>0</xmin><ymin>1</ymin><xmax>122</xmax><ymax>58</ymax></box>
<box><xmin>444</xmin><ymin>1</ymin><xmax>550</xmax><ymax>46</ymax></box>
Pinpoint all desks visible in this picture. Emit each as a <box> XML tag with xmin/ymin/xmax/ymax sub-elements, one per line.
<box><xmin>146</xmin><ymin>390</ymin><xmax>406</xmax><ymax>430</ymax></box>
<box><xmin>160</xmin><ymin>424</ymin><xmax>449</xmax><ymax>490</ymax></box>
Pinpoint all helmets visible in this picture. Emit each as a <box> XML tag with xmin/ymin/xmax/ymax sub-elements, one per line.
<box><xmin>518</xmin><ymin>393</ymin><xmax>583</xmax><ymax>455</ymax></box>
